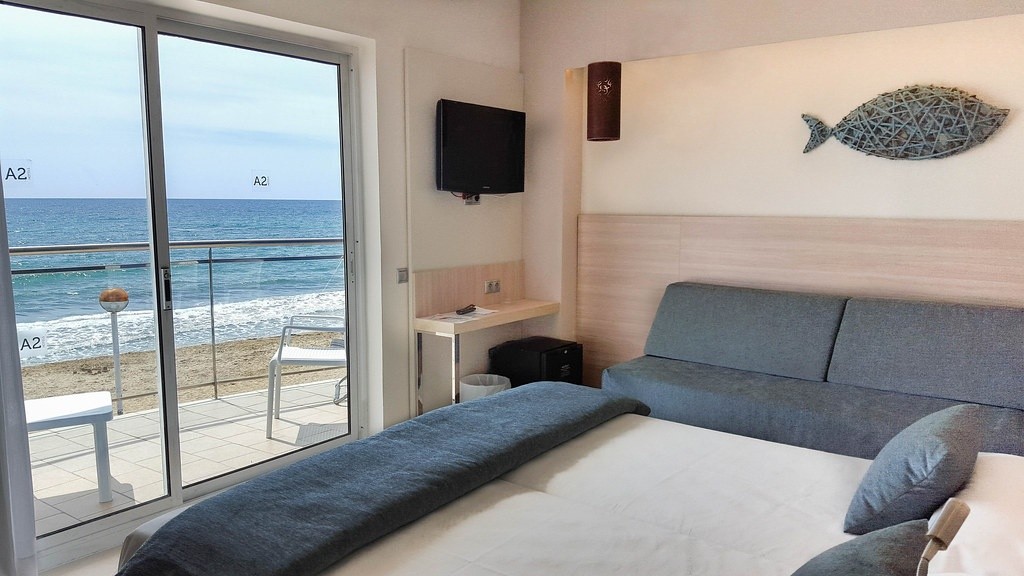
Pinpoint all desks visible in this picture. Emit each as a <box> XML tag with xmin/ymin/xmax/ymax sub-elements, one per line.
<box><xmin>416</xmin><ymin>298</ymin><xmax>559</xmax><ymax>417</ymax></box>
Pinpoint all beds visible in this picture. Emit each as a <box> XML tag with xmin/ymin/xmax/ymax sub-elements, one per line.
<box><xmin>117</xmin><ymin>380</ymin><xmax>1024</xmax><ymax>576</ymax></box>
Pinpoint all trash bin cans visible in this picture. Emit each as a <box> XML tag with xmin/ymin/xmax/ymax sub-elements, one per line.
<box><xmin>459</xmin><ymin>373</ymin><xmax>510</xmax><ymax>405</ymax></box>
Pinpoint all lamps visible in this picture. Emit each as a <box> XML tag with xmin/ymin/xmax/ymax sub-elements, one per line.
<box><xmin>587</xmin><ymin>62</ymin><xmax>621</xmax><ymax>141</ymax></box>
<box><xmin>916</xmin><ymin>497</ymin><xmax>971</xmax><ymax>576</ymax></box>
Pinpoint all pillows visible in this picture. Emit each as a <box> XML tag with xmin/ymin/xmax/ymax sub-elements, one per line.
<box><xmin>844</xmin><ymin>401</ymin><xmax>981</xmax><ymax>536</ymax></box>
<box><xmin>790</xmin><ymin>517</ymin><xmax>927</xmax><ymax>576</ymax></box>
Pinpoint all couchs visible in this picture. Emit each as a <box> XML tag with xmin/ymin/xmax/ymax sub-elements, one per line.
<box><xmin>600</xmin><ymin>282</ymin><xmax>1024</xmax><ymax>463</ymax></box>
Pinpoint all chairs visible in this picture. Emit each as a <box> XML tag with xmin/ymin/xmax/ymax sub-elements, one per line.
<box><xmin>266</xmin><ymin>315</ymin><xmax>345</xmax><ymax>438</ymax></box>
<box><xmin>25</xmin><ymin>390</ymin><xmax>113</xmax><ymax>502</ymax></box>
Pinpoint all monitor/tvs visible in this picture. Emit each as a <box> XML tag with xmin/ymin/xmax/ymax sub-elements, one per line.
<box><xmin>435</xmin><ymin>99</ymin><xmax>526</xmax><ymax>194</ymax></box>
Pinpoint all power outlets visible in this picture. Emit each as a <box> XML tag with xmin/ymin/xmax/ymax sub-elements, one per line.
<box><xmin>485</xmin><ymin>280</ymin><xmax>501</xmax><ymax>294</ymax></box>
